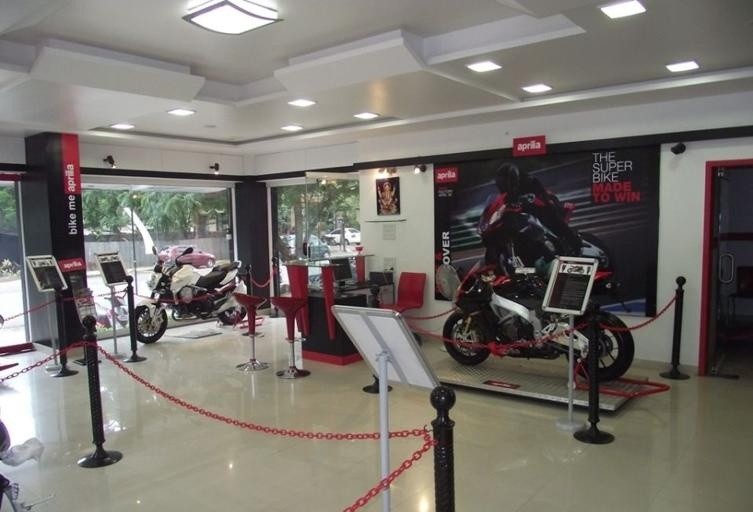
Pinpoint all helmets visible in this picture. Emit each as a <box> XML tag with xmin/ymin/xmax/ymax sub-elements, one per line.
<box><xmin>494</xmin><ymin>162</ymin><xmax>522</xmax><ymax>194</ymax></box>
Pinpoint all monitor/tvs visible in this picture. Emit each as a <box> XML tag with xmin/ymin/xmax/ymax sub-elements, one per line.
<box><xmin>330</xmin><ymin>257</ymin><xmax>354</xmax><ymax>289</ymax></box>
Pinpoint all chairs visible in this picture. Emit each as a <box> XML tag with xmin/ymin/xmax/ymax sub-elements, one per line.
<box><xmin>726</xmin><ymin>265</ymin><xmax>753</xmax><ymax>323</ymax></box>
<box><xmin>378</xmin><ymin>272</ymin><xmax>426</xmax><ymax>313</ymax></box>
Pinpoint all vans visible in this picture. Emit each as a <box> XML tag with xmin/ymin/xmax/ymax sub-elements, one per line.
<box><xmin>280</xmin><ymin>234</ymin><xmax>330</xmax><ymax>260</ymax></box>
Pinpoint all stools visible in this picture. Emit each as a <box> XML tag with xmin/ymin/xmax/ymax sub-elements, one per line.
<box><xmin>271</xmin><ymin>297</ymin><xmax>311</xmax><ymax>379</ymax></box>
<box><xmin>232</xmin><ymin>292</ymin><xmax>270</xmax><ymax>372</ymax></box>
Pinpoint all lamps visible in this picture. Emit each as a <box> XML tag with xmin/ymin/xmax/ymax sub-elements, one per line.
<box><xmin>103</xmin><ymin>156</ymin><xmax>116</xmax><ymax>168</ymax></box>
<box><xmin>210</xmin><ymin>163</ymin><xmax>219</xmax><ymax>176</ymax></box>
<box><xmin>415</xmin><ymin>165</ymin><xmax>426</xmax><ymax>174</ymax></box>
<box><xmin>181</xmin><ymin>0</ymin><xmax>284</xmax><ymax>35</ymax></box>
<box><xmin>671</xmin><ymin>143</ymin><xmax>685</xmax><ymax>155</ymax></box>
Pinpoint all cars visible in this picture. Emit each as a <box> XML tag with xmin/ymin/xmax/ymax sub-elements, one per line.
<box><xmin>158</xmin><ymin>244</ymin><xmax>217</xmax><ymax>268</ymax></box>
<box><xmin>323</xmin><ymin>227</ymin><xmax>360</xmax><ymax>245</ymax></box>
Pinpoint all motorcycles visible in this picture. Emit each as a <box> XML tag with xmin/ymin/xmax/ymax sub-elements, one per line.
<box><xmin>320</xmin><ymin>235</ymin><xmax>336</xmax><ymax>246</ymax></box>
<box><xmin>477</xmin><ymin>191</ymin><xmax>618</xmax><ymax>292</ymax></box>
<box><xmin>91</xmin><ymin>291</ymin><xmax>128</xmax><ymax>330</ymax></box>
<box><xmin>442</xmin><ymin>253</ymin><xmax>633</xmax><ymax>384</ymax></box>
<box><xmin>133</xmin><ymin>246</ymin><xmax>248</xmax><ymax>344</ymax></box>
